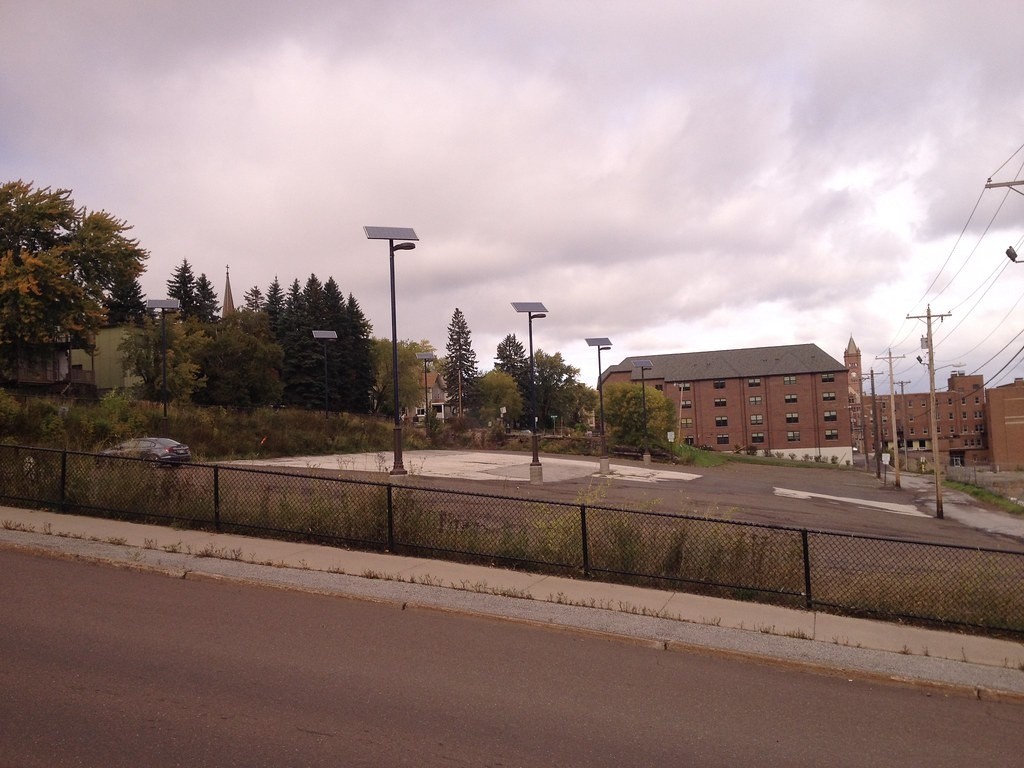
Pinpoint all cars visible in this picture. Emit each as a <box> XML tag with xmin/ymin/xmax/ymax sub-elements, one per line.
<box><xmin>97</xmin><ymin>437</ymin><xmax>192</xmax><ymax>470</ymax></box>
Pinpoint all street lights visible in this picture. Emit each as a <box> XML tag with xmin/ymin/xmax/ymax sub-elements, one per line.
<box><xmin>363</xmin><ymin>225</ymin><xmax>420</xmax><ymax>475</ymax></box>
<box><xmin>584</xmin><ymin>338</ymin><xmax>613</xmax><ymax>475</ymax></box>
<box><xmin>313</xmin><ymin>330</ymin><xmax>338</xmax><ymax>418</ymax></box>
<box><xmin>145</xmin><ymin>298</ymin><xmax>180</xmax><ymax>438</ymax></box>
<box><xmin>631</xmin><ymin>359</ymin><xmax>653</xmax><ymax>466</ymax></box>
<box><xmin>511</xmin><ymin>301</ymin><xmax>549</xmax><ymax>486</ymax></box>
<box><xmin>415</xmin><ymin>351</ymin><xmax>436</xmax><ymax>426</ymax></box>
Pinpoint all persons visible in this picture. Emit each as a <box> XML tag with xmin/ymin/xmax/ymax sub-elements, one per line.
<box><xmin>400</xmin><ymin>411</ymin><xmax>419</xmax><ymax>423</ymax></box>
